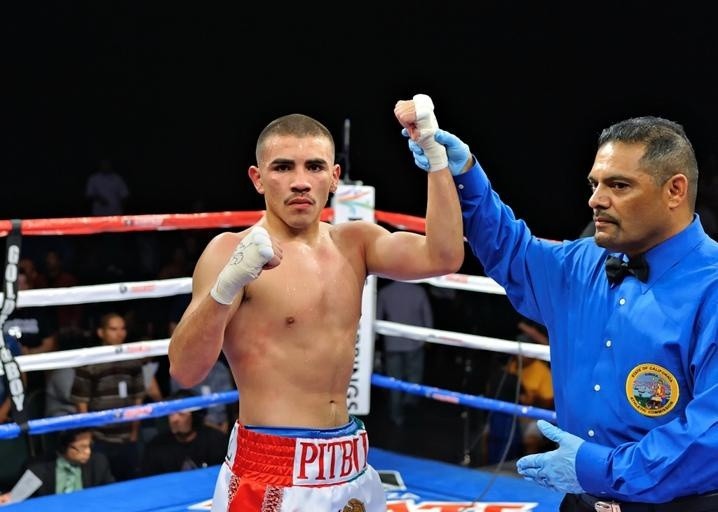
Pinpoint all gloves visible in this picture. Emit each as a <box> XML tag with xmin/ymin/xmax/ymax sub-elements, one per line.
<box><xmin>401</xmin><ymin>127</ymin><xmax>471</xmax><ymax>178</ymax></box>
<box><xmin>515</xmin><ymin>419</ymin><xmax>586</xmax><ymax>495</ymax></box>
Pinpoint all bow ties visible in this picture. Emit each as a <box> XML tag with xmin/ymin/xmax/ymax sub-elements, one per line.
<box><xmin>604</xmin><ymin>255</ymin><xmax>650</xmax><ymax>287</ymax></box>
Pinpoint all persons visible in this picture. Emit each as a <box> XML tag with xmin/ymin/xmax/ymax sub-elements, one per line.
<box><xmin>375</xmin><ymin>273</ymin><xmax>554</xmax><ymax>464</ymax></box>
<box><xmin>87</xmin><ymin>156</ymin><xmax>133</xmax><ymax>217</ymax></box>
<box><xmin>1</xmin><ymin>238</ymin><xmax>237</xmax><ymax>505</ymax></box>
<box><xmin>168</xmin><ymin>92</ymin><xmax>465</xmax><ymax>512</ymax></box>
<box><xmin>402</xmin><ymin>116</ymin><xmax>718</xmax><ymax>512</ymax></box>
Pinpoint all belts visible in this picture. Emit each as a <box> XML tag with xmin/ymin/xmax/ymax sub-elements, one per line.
<box><xmin>581</xmin><ymin>493</ymin><xmax>642</xmax><ymax>512</ymax></box>
